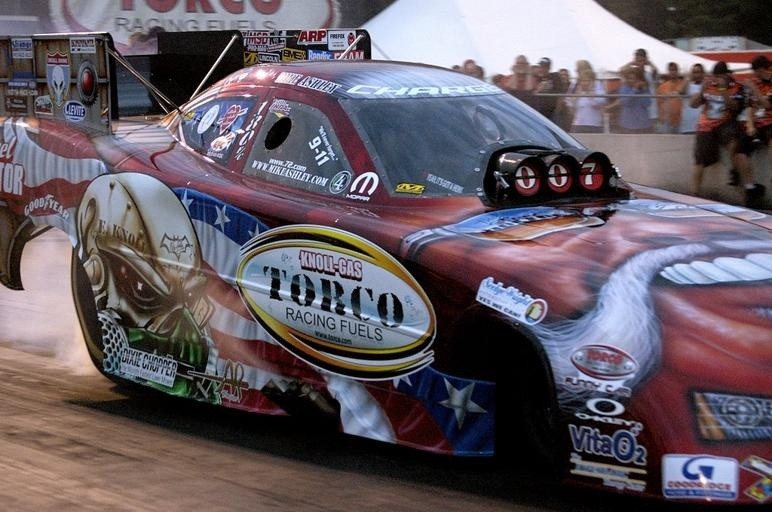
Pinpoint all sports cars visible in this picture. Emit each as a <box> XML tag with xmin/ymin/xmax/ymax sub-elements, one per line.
<box><xmin>0</xmin><ymin>58</ymin><xmax>771</xmax><ymax>511</ymax></box>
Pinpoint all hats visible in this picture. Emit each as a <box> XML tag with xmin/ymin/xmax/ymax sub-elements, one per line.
<box><xmin>714</xmin><ymin>60</ymin><xmax>733</xmax><ymax>74</ymax></box>
<box><xmin>538</xmin><ymin>57</ymin><xmax>550</xmax><ymax>65</ymax></box>
<box><xmin>635</xmin><ymin>49</ymin><xmax>646</xmax><ymax>55</ymax></box>
<box><xmin>750</xmin><ymin>56</ymin><xmax>771</xmax><ymax>69</ymax></box>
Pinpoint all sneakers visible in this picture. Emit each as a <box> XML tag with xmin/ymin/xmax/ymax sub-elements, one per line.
<box><xmin>727</xmin><ymin>167</ymin><xmax>741</xmax><ymax>186</ymax></box>
<box><xmin>745</xmin><ymin>183</ymin><xmax>765</xmax><ymax>207</ymax></box>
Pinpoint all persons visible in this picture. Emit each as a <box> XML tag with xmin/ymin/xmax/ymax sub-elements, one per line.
<box><xmin>453</xmin><ymin>48</ymin><xmax>705</xmax><ymax>133</ymax></box>
<box><xmin>690</xmin><ymin>56</ymin><xmax>772</xmax><ymax>210</ymax></box>
